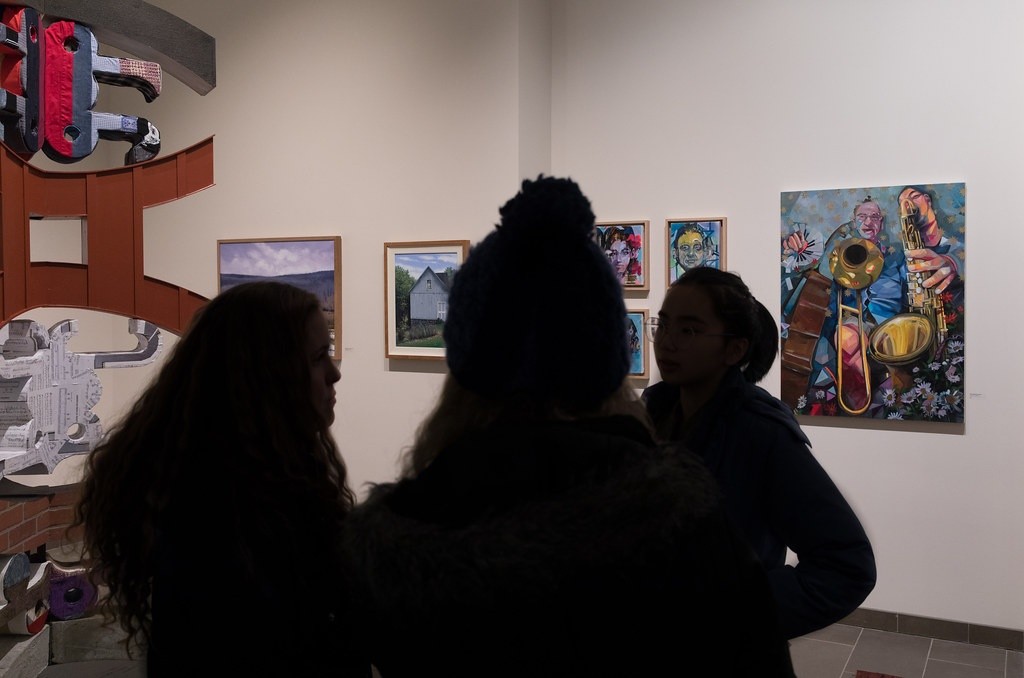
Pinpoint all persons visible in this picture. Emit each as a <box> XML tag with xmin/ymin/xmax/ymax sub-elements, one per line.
<box><xmin>640</xmin><ymin>266</ymin><xmax>877</xmax><ymax>678</ymax></box>
<box><xmin>82</xmin><ymin>282</ymin><xmax>373</xmax><ymax>677</ymax></box>
<box><xmin>317</xmin><ymin>180</ymin><xmax>793</xmax><ymax>678</ymax></box>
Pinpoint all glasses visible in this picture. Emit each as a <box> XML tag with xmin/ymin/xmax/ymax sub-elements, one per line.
<box><xmin>644</xmin><ymin>319</ymin><xmax>736</xmax><ymax>349</ymax></box>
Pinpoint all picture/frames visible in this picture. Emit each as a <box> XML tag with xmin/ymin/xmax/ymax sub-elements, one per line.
<box><xmin>383</xmin><ymin>240</ymin><xmax>470</xmax><ymax>361</ymax></box>
<box><xmin>625</xmin><ymin>309</ymin><xmax>650</xmax><ymax>380</ymax></box>
<box><xmin>664</xmin><ymin>217</ymin><xmax>727</xmax><ymax>290</ymax></box>
<box><xmin>216</xmin><ymin>235</ymin><xmax>341</xmax><ymax>360</ymax></box>
<box><xmin>594</xmin><ymin>220</ymin><xmax>651</xmax><ymax>292</ymax></box>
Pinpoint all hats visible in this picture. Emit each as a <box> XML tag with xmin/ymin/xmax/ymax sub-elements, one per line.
<box><xmin>444</xmin><ymin>173</ymin><xmax>632</xmax><ymax>416</ymax></box>
